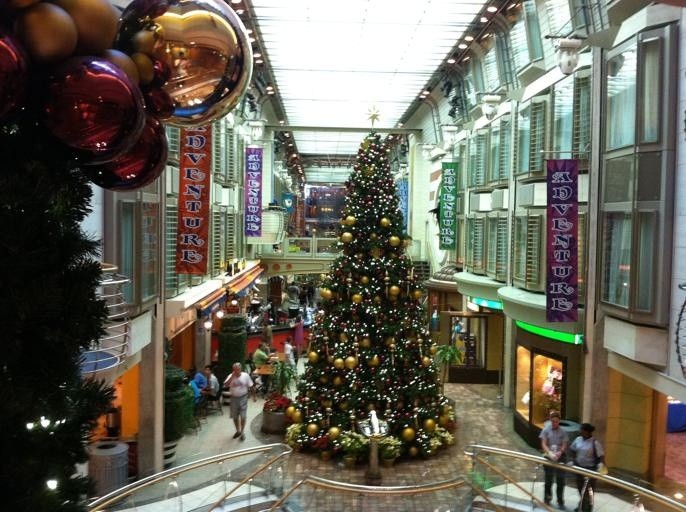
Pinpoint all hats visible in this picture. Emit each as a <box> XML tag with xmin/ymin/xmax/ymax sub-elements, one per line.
<box><xmin>580</xmin><ymin>422</ymin><xmax>596</xmax><ymax>434</ymax></box>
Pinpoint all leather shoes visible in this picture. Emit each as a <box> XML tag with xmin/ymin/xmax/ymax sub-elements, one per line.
<box><xmin>233</xmin><ymin>432</ymin><xmax>241</xmax><ymax>438</ymax></box>
<box><xmin>240</xmin><ymin>434</ymin><xmax>246</xmax><ymax>440</ymax></box>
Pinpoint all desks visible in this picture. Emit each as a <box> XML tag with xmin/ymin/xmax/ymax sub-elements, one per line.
<box><xmin>251</xmin><ymin>352</ymin><xmax>285</xmax><ymax>395</ymax></box>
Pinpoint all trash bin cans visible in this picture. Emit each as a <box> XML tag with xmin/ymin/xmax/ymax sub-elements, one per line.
<box><xmin>85</xmin><ymin>440</ymin><xmax>129</xmax><ymax>508</ymax></box>
<box><xmin>543</xmin><ymin>419</ymin><xmax>581</xmax><ymax>463</ymax></box>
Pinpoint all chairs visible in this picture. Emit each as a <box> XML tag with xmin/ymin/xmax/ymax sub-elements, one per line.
<box><xmin>204</xmin><ymin>382</ymin><xmax>223</xmax><ymax>417</ymax></box>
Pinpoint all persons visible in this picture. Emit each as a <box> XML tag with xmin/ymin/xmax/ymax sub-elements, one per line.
<box><xmin>182</xmin><ymin>365</ymin><xmax>221</xmax><ymax>419</ymax></box>
<box><xmin>539</xmin><ymin>412</ymin><xmax>569</xmax><ymax>505</ymax></box>
<box><xmin>570</xmin><ymin>424</ymin><xmax>604</xmax><ymax>512</ymax></box>
<box><xmin>242</xmin><ymin>274</ymin><xmax>329</xmax><ymax>399</ymax></box>
<box><xmin>542</xmin><ymin>366</ymin><xmax>563</xmax><ymax>420</ymax></box>
<box><xmin>223</xmin><ymin>361</ymin><xmax>257</xmax><ymax>441</ymax></box>
<box><xmin>451</xmin><ymin>316</ymin><xmax>466</xmax><ymax>363</ymax></box>
